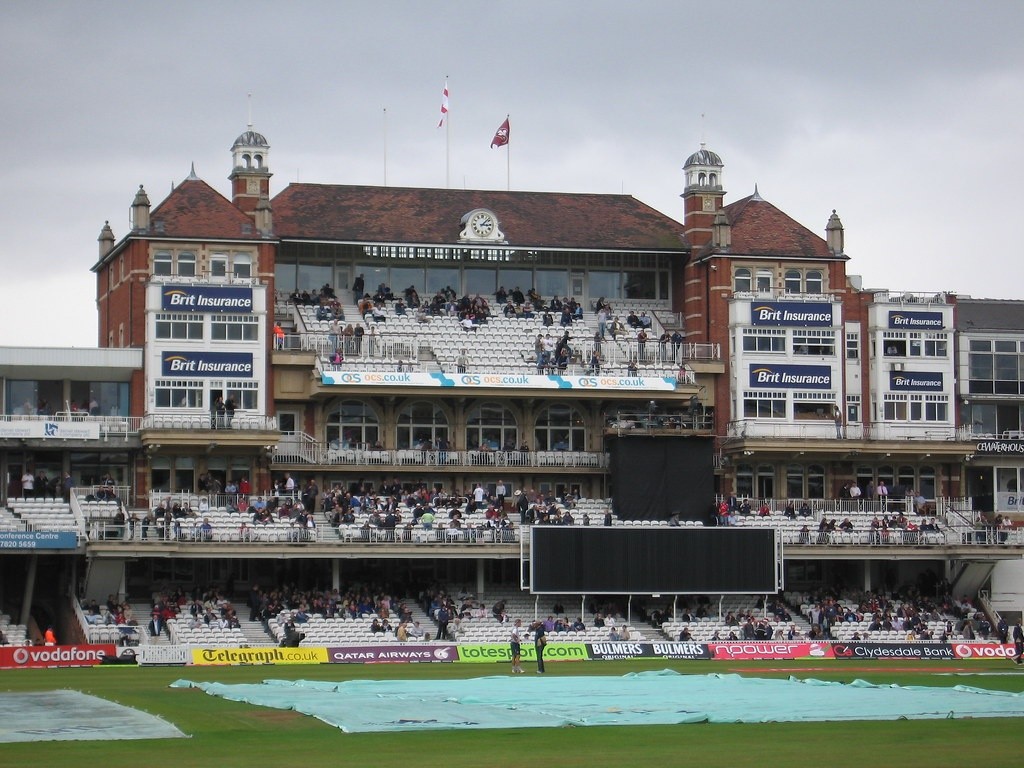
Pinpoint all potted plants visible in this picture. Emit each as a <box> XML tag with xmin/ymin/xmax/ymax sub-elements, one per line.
<box><xmin>945</xmin><ymin>290</ymin><xmax>958</xmax><ymax>304</ymax></box>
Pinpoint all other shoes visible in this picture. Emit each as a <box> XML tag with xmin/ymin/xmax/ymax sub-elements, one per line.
<box><xmin>1011</xmin><ymin>657</ymin><xmax>1017</xmax><ymax>664</ymax></box>
<box><xmin>1018</xmin><ymin>663</ymin><xmax>1024</xmax><ymax>666</ymax></box>
<box><xmin>536</xmin><ymin>670</ymin><xmax>541</xmax><ymax>673</ymax></box>
<box><xmin>512</xmin><ymin>666</ymin><xmax>524</xmax><ymax>674</ymax></box>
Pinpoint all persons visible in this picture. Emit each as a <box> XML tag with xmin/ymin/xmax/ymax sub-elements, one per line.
<box><xmin>510</xmin><ymin>619</ymin><xmax>525</xmax><ymax>674</ymax></box>
<box><xmin>535</xmin><ymin>618</ymin><xmax>547</xmax><ymax>674</ymax></box>
<box><xmin>17</xmin><ymin>273</ymin><xmax>1023</xmax><ymax>667</ymax></box>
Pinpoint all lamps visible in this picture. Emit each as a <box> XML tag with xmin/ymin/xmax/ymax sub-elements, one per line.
<box><xmin>856</xmin><ymin>359</ymin><xmax>862</xmax><ymax>366</ymax></box>
<box><xmin>964</xmin><ymin>400</ymin><xmax>969</xmax><ymax>405</ymax></box>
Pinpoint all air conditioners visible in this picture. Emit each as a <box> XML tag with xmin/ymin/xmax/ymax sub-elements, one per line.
<box><xmin>711</xmin><ymin>265</ymin><xmax>717</xmax><ymax>271</ymax></box>
<box><xmin>891</xmin><ymin>362</ymin><xmax>904</xmax><ymax>371</ymax></box>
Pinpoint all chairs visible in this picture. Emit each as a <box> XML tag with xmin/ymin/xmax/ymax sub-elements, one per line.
<box><xmin>273</xmin><ymin>291</ymin><xmax>682</xmax><ymax>377</ymax></box>
<box><xmin>330</xmin><ymin>447</ymin><xmax>598</xmax><ymax>466</ymax></box>
<box><xmin>0</xmin><ymin>495</ymin><xmax>118</xmax><ymax>534</ymax></box>
<box><xmin>145</xmin><ymin>416</ymin><xmax>276</xmax><ymax>430</ymax></box>
<box><xmin>0</xmin><ymin>591</ymin><xmax>1001</xmax><ymax>646</ymax></box>
<box><xmin>159</xmin><ymin>505</ymin><xmax>1024</xmax><ymax>540</ymax></box>
<box><xmin>13</xmin><ymin>414</ymin><xmax>129</xmax><ymax>432</ymax></box>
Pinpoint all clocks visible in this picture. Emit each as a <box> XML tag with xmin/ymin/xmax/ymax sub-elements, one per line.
<box><xmin>471</xmin><ymin>213</ymin><xmax>495</xmax><ymax>237</ymax></box>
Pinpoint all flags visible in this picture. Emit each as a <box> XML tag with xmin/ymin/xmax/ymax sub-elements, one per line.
<box><xmin>490</xmin><ymin>118</ymin><xmax>510</xmax><ymax>148</ymax></box>
<box><xmin>436</xmin><ymin>89</ymin><xmax>449</xmax><ymax>129</ymax></box>
